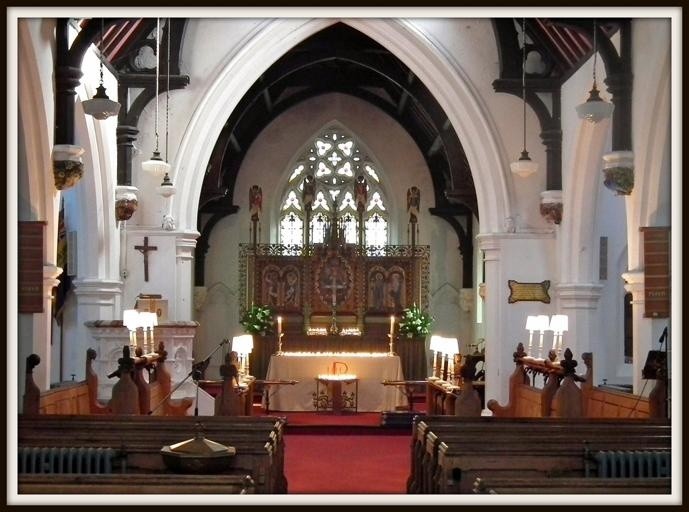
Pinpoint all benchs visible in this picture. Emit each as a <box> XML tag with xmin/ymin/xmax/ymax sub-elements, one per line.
<box><xmin>404</xmin><ymin>411</ymin><xmax>671</xmax><ymax>494</ymax></box>
<box><xmin>18</xmin><ymin>407</ymin><xmax>287</xmax><ymax>496</ymax></box>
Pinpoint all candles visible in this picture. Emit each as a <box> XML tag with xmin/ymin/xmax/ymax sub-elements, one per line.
<box><xmin>277</xmin><ymin>320</ymin><xmax>282</xmax><ymax>334</ymax></box>
<box><xmin>390</xmin><ymin>319</ymin><xmax>395</xmax><ymax>335</ymax></box>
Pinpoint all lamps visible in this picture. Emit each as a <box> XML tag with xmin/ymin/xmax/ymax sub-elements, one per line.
<box><xmin>427</xmin><ymin>333</ymin><xmax>462</xmax><ymax>393</ymax></box>
<box><xmin>230</xmin><ymin>333</ymin><xmax>255</xmax><ymax>390</ymax></box>
<box><xmin>508</xmin><ymin>19</ymin><xmax>618</xmax><ymax>181</ymax></box>
<box><xmin>524</xmin><ymin>314</ymin><xmax>569</xmax><ymax>366</ymax></box>
<box><xmin>121</xmin><ymin>309</ymin><xmax>159</xmax><ymax>359</ymax></box>
<box><xmin>81</xmin><ymin>17</ymin><xmax>179</xmax><ymax>202</ymax></box>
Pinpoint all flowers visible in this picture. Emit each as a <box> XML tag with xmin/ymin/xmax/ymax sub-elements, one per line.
<box><xmin>237</xmin><ymin>297</ymin><xmax>275</xmax><ymax>335</ymax></box>
<box><xmin>400</xmin><ymin>301</ymin><xmax>439</xmax><ymax>342</ymax></box>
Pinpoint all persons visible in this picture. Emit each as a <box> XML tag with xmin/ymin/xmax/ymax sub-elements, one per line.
<box><xmin>354</xmin><ymin>175</ymin><xmax>368</xmax><ymax>209</ymax></box>
<box><xmin>407</xmin><ymin>185</ymin><xmax>419</xmax><ymax>223</ymax></box>
<box><xmin>248</xmin><ymin>184</ymin><xmax>262</xmax><ymax>218</ymax></box>
<box><xmin>301</xmin><ymin>175</ymin><xmax>315</xmax><ymax>208</ymax></box>
